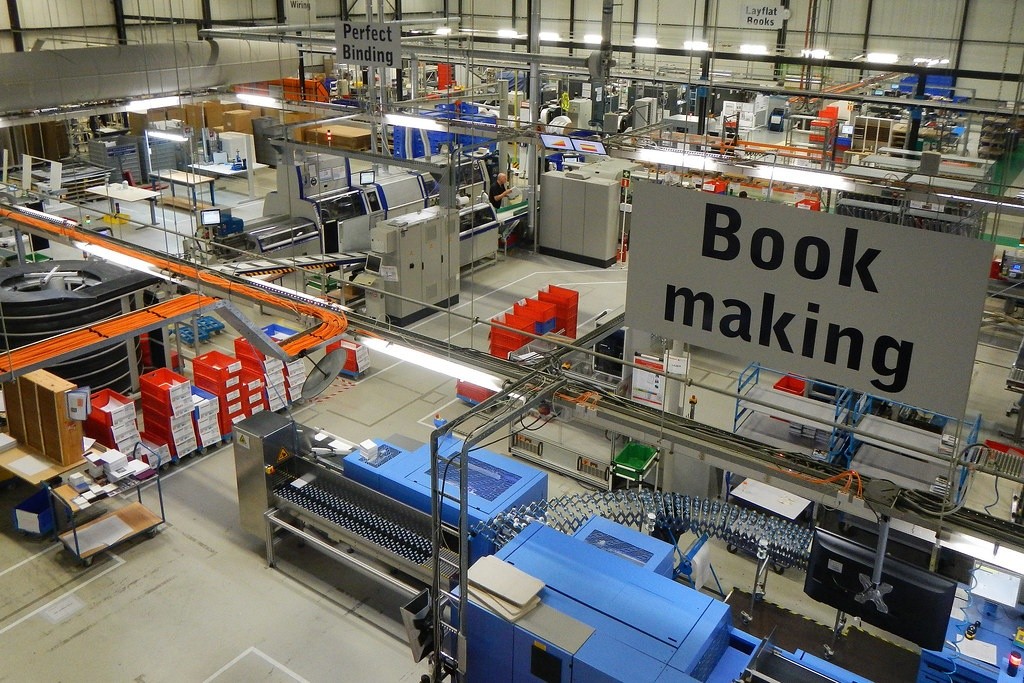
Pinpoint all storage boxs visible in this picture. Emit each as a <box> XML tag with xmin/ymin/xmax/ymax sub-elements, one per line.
<box><xmin>83</xmin><ymin>323</ymin><xmax>371</xmax><ymax>464</ymax></box>
<box><xmin>615</xmin><ymin>442</ymin><xmax>656</xmax><ymax>479</ymax></box>
<box><xmin>809</xmin><ymin>106</ymin><xmax>851</xmax><ymax>164</ymax></box>
<box><xmin>456</xmin><ymin>286</ymin><xmax>579</xmax><ymax>404</ymax></box>
<box><xmin>768</xmin><ymin>373</ymin><xmax>805</xmax><ymax>423</ymax></box>
<box><xmin>26</xmin><ymin>251</ymin><xmax>52</xmax><ymax>265</ymax></box>
<box><xmin>795</xmin><ymin>198</ymin><xmax>821</xmax><ymax>211</ymax></box>
<box><xmin>703</xmin><ymin>180</ymin><xmax>726</xmax><ymax>193</ymax></box>
<box><xmin>101</xmin><ymin>213</ymin><xmax>129</xmax><ymax>225</ymax></box>
<box><xmin>213</xmin><ymin>109</ymin><xmax>254</xmax><ymax>135</ymax></box>
<box><xmin>395</xmin><ymin>101</ymin><xmax>497</xmax><ymax>161</ymax></box>
<box><xmin>12</xmin><ymin>489</ymin><xmax>75</xmax><ymax>536</ymax></box>
<box><xmin>985</xmin><ymin>440</ymin><xmax>1024</xmax><ymax>458</ymax></box>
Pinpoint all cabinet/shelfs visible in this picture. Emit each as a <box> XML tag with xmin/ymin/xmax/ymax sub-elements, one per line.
<box><xmin>783</xmin><ymin>114</ymin><xmax>833</xmax><ymax>166</ymax></box>
<box><xmin>374</xmin><ymin>206</ymin><xmax>460</xmax><ymax>328</ymax></box>
<box><xmin>979</xmin><ymin>121</ymin><xmax>1007</xmax><ymax>159</ymax></box>
<box><xmin>40</xmin><ymin>441</ymin><xmax>166</xmax><ymax>568</ymax></box>
<box><xmin>2</xmin><ymin>368</ymin><xmax>84</xmax><ymax>467</ymax></box>
<box><xmin>725</xmin><ymin>361</ymin><xmax>984</xmax><ymax>530</ymax></box>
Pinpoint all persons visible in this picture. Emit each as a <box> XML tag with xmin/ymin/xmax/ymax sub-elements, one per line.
<box><xmin>738</xmin><ymin>191</ymin><xmax>747</xmax><ymax>199</ymax></box>
<box><xmin>341</xmin><ymin>73</ymin><xmax>351</xmax><ymax>99</ymax></box>
<box><xmin>489</xmin><ymin>173</ymin><xmax>516</xmax><ymax>209</ymax></box>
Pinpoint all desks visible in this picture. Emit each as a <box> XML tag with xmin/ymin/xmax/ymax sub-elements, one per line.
<box><xmin>841</xmin><ymin>145</ymin><xmax>997</xmax><ymax>192</ymax></box>
<box><xmin>84</xmin><ymin>182</ymin><xmax>164</xmax><ymax>231</ymax></box>
<box><xmin>727</xmin><ymin>478</ymin><xmax>811</xmax><ymax>574</ymax></box>
<box><xmin>0</xmin><ymin>424</ymin><xmax>110</xmax><ymax>543</ymax></box>
<box><xmin>892</xmin><ymin>122</ymin><xmax>949</xmax><ymax>152</ymax></box>
<box><xmin>187</xmin><ymin>128</ymin><xmax>269</xmax><ymax>204</ymax></box>
<box><xmin>147</xmin><ymin>169</ymin><xmax>216</xmax><ymax>215</ymax></box>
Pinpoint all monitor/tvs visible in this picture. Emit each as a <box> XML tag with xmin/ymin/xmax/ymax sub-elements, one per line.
<box><xmin>970</xmin><ymin>559</ymin><xmax>1024</xmax><ymax>610</ymax></box>
<box><xmin>541</xmin><ymin>134</ymin><xmax>575</xmax><ymax>151</ymax></box>
<box><xmin>571</xmin><ymin>138</ymin><xmax>607</xmax><ymax>155</ymax></box>
<box><xmin>802</xmin><ymin>525</ymin><xmax>958</xmax><ymax>653</ymax></box>
<box><xmin>360</xmin><ymin>170</ymin><xmax>375</xmax><ymax>185</ymax></box>
<box><xmin>365</xmin><ymin>254</ymin><xmax>382</xmax><ymax>274</ymax></box>
<box><xmin>200</xmin><ymin>209</ymin><xmax>221</xmax><ymax>226</ymax></box>
